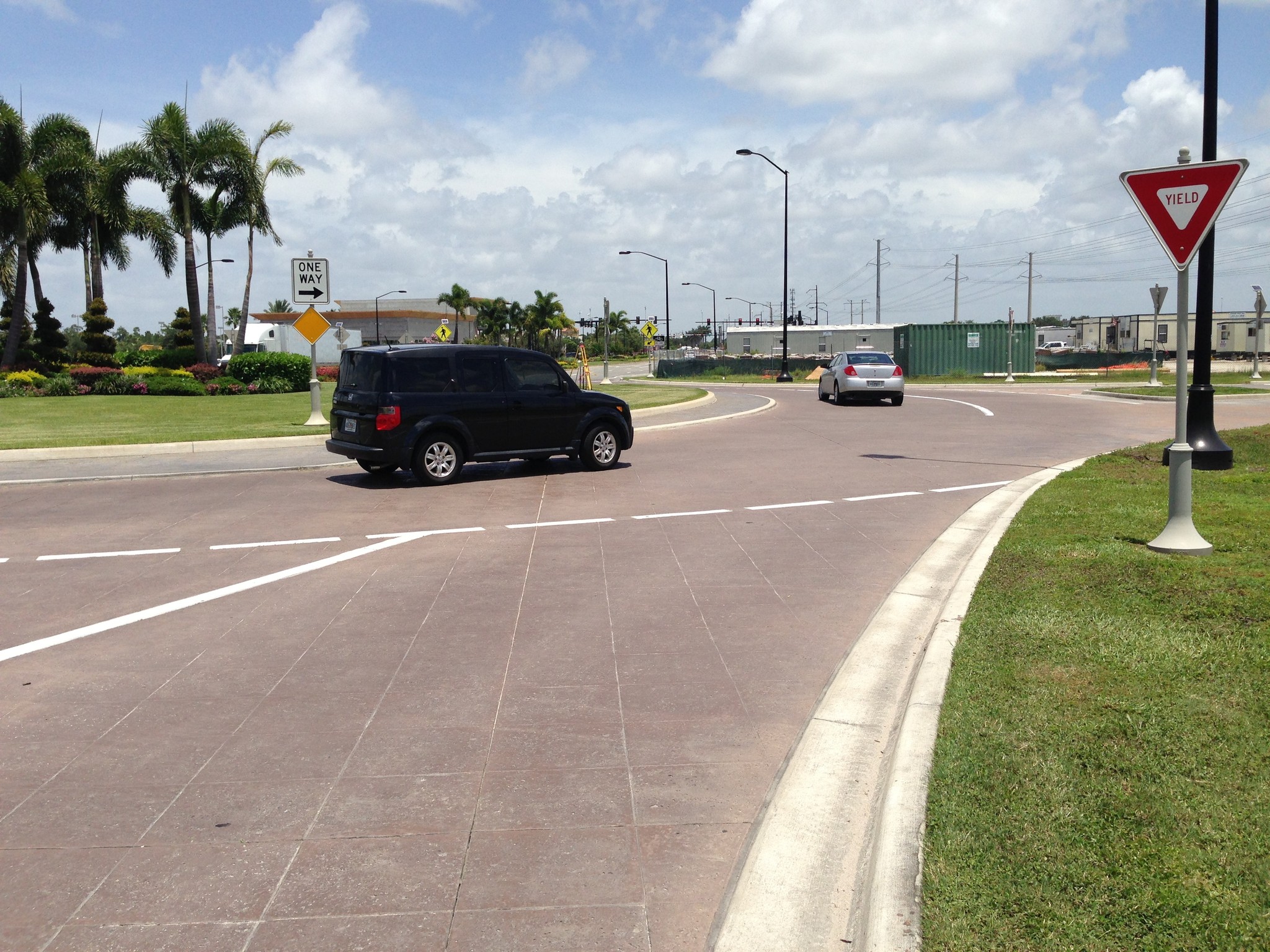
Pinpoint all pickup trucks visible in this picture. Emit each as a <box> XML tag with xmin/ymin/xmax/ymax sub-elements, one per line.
<box><xmin>1035</xmin><ymin>341</ymin><xmax>1075</xmax><ymax>350</ymax></box>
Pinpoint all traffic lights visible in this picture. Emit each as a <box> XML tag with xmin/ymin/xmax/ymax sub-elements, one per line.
<box><xmin>739</xmin><ymin>318</ymin><xmax>742</xmax><ymax>325</ymax></box>
<box><xmin>707</xmin><ymin>319</ymin><xmax>710</xmax><ymax>325</ymax></box>
<box><xmin>756</xmin><ymin>318</ymin><xmax>759</xmax><ymax>325</ymax></box>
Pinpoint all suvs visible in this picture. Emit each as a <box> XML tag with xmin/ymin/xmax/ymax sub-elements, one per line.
<box><xmin>325</xmin><ymin>343</ymin><xmax>634</xmax><ymax>486</ymax></box>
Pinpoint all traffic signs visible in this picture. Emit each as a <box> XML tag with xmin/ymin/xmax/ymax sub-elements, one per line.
<box><xmin>291</xmin><ymin>257</ymin><xmax>330</xmax><ymax>305</ymax></box>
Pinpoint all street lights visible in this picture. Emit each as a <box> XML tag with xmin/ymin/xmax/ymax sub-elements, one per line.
<box><xmin>809</xmin><ymin>307</ymin><xmax>828</xmax><ymax>325</ymax></box>
<box><xmin>725</xmin><ymin>298</ymin><xmax>751</xmax><ymax>326</ymax></box>
<box><xmin>619</xmin><ymin>250</ymin><xmax>670</xmax><ymax>360</ymax></box>
<box><xmin>795</xmin><ymin>314</ymin><xmax>811</xmax><ymax>325</ymax></box>
<box><xmin>682</xmin><ymin>282</ymin><xmax>717</xmax><ymax>353</ymax></box>
<box><xmin>215</xmin><ymin>305</ymin><xmax>227</xmax><ymax>358</ymax></box>
<box><xmin>583</xmin><ymin>316</ymin><xmax>598</xmax><ymax>344</ymax></box>
<box><xmin>750</xmin><ymin>302</ymin><xmax>773</xmax><ymax>325</ymax></box>
<box><xmin>735</xmin><ymin>149</ymin><xmax>792</xmax><ymax>382</ymax></box>
<box><xmin>376</xmin><ymin>290</ymin><xmax>407</xmax><ymax>345</ymax></box>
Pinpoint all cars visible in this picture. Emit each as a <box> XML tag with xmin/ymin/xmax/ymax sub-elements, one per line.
<box><xmin>818</xmin><ymin>350</ymin><xmax>905</xmax><ymax>406</ymax></box>
